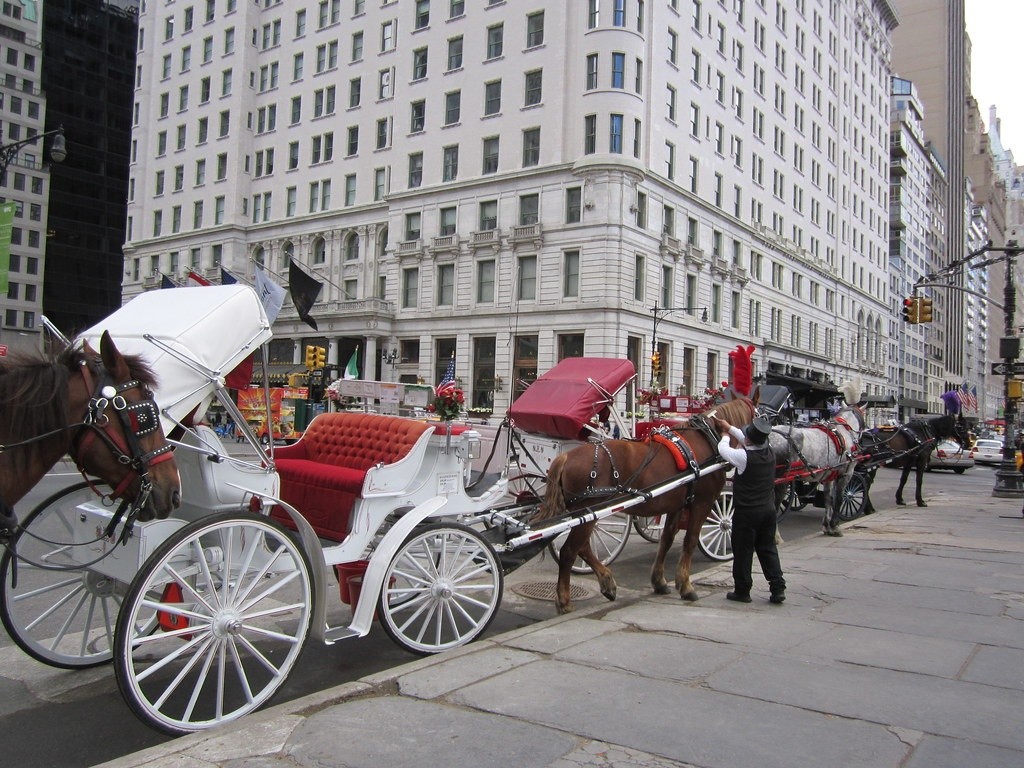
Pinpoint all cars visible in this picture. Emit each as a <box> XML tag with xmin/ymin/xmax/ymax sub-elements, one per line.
<box><xmin>928</xmin><ymin>440</ymin><xmax>974</xmax><ymax>474</ymax></box>
<box><xmin>974</xmin><ymin>438</ymin><xmax>1004</xmax><ymax>464</ymax></box>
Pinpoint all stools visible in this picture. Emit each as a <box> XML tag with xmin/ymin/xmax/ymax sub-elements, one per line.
<box><xmin>426</xmin><ymin>420</ymin><xmax>471</xmax><ymax>448</ymax></box>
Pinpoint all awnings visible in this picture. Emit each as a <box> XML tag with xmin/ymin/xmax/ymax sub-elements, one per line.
<box><xmin>767</xmin><ymin>369</ymin><xmax>838</xmax><ymax>405</ymax></box>
<box><xmin>859</xmin><ymin>396</ymin><xmax>895</xmax><ymax>408</ymax></box>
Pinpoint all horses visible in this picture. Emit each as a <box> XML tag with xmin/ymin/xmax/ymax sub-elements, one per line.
<box><xmin>765</xmin><ymin>399</ymin><xmax>870</xmax><ymax>544</ymax></box>
<box><xmin>0</xmin><ymin>330</ymin><xmax>180</xmax><ymax>540</ymax></box>
<box><xmin>535</xmin><ymin>385</ymin><xmax>761</xmax><ymax>615</ymax></box>
<box><xmin>850</xmin><ymin>411</ymin><xmax>971</xmax><ymax>514</ymax></box>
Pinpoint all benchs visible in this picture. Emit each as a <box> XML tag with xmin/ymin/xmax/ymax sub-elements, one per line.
<box><xmin>168</xmin><ymin>425</ymin><xmax>279</xmax><ymax>511</ymax></box>
<box><xmin>263</xmin><ymin>413</ymin><xmax>435</xmax><ymax>540</ymax></box>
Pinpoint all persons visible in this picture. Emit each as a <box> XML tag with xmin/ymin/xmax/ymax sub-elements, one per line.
<box><xmin>605</xmin><ymin>419</ymin><xmax>640</xmax><ymax>440</ymax></box>
<box><xmin>716</xmin><ymin>418</ymin><xmax>787</xmax><ymax>603</ymax></box>
<box><xmin>967</xmin><ymin>425</ymin><xmax>1024</xmax><ymax>451</ymax></box>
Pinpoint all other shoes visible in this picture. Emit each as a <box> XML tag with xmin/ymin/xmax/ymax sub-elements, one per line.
<box><xmin>770</xmin><ymin>590</ymin><xmax>786</xmax><ymax>603</ymax></box>
<box><xmin>727</xmin><ymin>590</ymin><xmax>753</xmax><ymax>602</ymax></box>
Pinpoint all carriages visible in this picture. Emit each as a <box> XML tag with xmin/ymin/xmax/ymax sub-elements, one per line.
<box><xmin>714</xmin><ymin>361</ymin><xmax>972</xmax><ymax>545</ymax></box>
<box><xmin>501</xmin><ymin>339</ymin><xmax>870</xmax><ymax>574</ymax></box>
<box><xmin>0</xmin><ymin>283</ymin><xmax>761</xmax><ymax>730</ymax></box>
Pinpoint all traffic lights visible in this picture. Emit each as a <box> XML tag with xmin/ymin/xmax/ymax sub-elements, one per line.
<box><xmin>306</xmin><ymin>348</ymin><xmax>327</xmax><ymax>371</ymax></box>
<box><xmin>900</xmin><ymin>299</ymin><xmax>912</xmax><ymax>323</ymax></box>
<box><xmin>920</xmin><ymin>298</ymin><xmax>933</xmax><ymax>322</ymax></box>
<box><xmin>652</xmin><ymin>352</ymin><xmax>661</xmax><ymax>376</ymax></box>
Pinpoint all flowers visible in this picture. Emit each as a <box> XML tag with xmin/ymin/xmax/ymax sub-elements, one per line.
<box><xmin>466</xmin><ymin>407</ymin><xmax>492</xmax><ymax>413</ymax></box>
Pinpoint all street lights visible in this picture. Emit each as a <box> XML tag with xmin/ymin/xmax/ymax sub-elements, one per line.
<box><xmin>649</xmin><ymin>300</ymin><xmax>708</xmax><ymax>386</ymax></box>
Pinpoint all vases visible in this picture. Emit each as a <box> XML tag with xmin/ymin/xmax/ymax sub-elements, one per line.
<box><xmin>467</xmin><ymin>411</ymin><xmax>493</xmax><ymax>419</ymax></box>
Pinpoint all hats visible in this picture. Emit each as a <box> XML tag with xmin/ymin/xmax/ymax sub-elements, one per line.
<box><xmin>741</xmin><ymin>417</ymin><xmax>772</xmax><ymax>449</ymax></box>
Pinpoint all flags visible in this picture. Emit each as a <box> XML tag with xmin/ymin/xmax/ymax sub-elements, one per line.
<box><xmin>957</xmin><ymin>382</ymin><xmax>1005</xmax><ymax>414</ymax></box>
<box><xmin>160</xmin><ymin>257</ymin><xmax>324</xmax><ymax>331</ymax></box>
<box><xmin>345</xmin><ymin>350</ymin><xmax>358</xmax><ymax>380</ymax></box>
<box><xmin>435</xmin><ymin>357</ymin><xmax>456</xmax><ymax>398</ymax></box>
